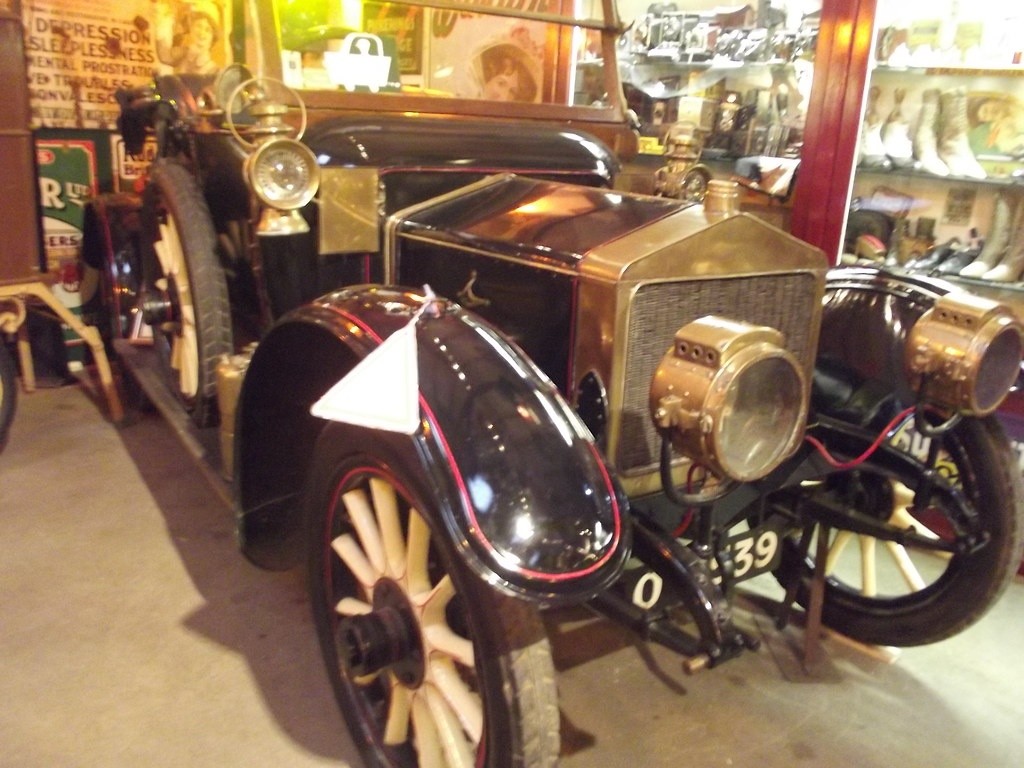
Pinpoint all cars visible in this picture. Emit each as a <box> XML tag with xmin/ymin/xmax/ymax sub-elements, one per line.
<box><xmin>73</xmin><ymin>1</ymin><xmax>1024</xmax><ymax>768</ymax></box>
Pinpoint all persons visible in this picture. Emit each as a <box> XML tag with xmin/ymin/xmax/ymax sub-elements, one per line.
<box><xmin>471</xmin><ymin>43</ymin><xmax>540</xmax><ymax>103</ymax></box>
<box><xmin>147</xmin><ymin>0</ymin><xmax>223</xmax><ymax>76</ymax></box>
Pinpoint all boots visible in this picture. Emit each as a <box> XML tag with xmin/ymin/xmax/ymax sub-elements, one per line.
<box><xmin>982</xmin><ymin>195</ymin><xmax>1024</xmax><ymax>282</ymax></box>
<box><xmin>904</xmin><ymin>217</ymin><xmax>936</xmax><ymax>268</ymax></box>
<box><xmin>960</xmin><ymin>192</ymin><xmax>1012</xmax><ymax>278</ymax></box>
<box><xmin>912</xmin><ymin>90</ymin><xmax>950</xmax><ymax>176</ymax></box>
<box><xmin>883</xmin><ymin>218</ymin><xmax>910</xmax><ymax>266</ymax></box>
<box><xmin>938</xmin><ymin>85</ymin><xmax>987</xmax><ymax>179</ymax></box>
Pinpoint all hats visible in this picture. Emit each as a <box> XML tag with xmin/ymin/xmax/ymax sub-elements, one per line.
<box><xmin>179</xmin><ymin>1</ymin><xmax>223</xmax><ymax>50</ymax></box>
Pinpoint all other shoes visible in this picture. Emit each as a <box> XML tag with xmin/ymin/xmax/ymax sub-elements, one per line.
<box><xmin>914</xmin><ymin>238</ymin><xmax>963</xmax><ymax>269</ymax></box>
<box><xmin>862</xmin><ymin>118</ymin><xmax>894</xmax><ymax>172</ymax></box>
<box><xmin>881</xmin><ymin>111</ymin><xmax>913</xmax><ymax>167</ymax></box>
<box><xmin>933</xmin><ymin>240</ymin><xmax>984</xmax><ymax>275</ymax></box>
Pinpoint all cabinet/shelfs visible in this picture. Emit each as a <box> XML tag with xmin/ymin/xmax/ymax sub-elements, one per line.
<box><xmin>573</xmin><ymin>0</ymin><xmax>1024</xmax><ymax>436</ymax></box>
